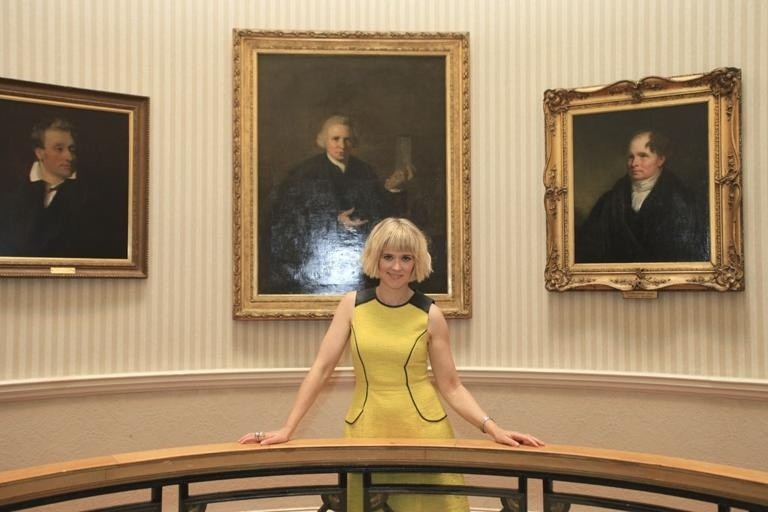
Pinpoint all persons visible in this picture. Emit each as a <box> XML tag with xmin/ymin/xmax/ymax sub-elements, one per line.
<box><xmin>4</xmin><ymin>115</ymin><xmax>110</xmax><ymax>257</ymax></box>
<box><xmin>574</xmin><ymin>126</ymin><xmax>706</xmax><ymax>260</ymax></box>
<box><xmin>270</xmin><ymin>111</ymin><xmax>415</xmax><ymax>295</ymax></box>
<box><xmin>236</xmin><ymin>215</ymin><xmax>546</xmax><ymax>511</ymax></box>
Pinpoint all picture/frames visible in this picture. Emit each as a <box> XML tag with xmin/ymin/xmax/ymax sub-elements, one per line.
<box><xmin>0</xmin><ymin>77</ymin><xmax>151</xmax><ymax>279</ymax></box>
<box><xmin>233</xmin><ymin>28</ymin><xmax>472</xmax><ymax>321</ymax></box>
<box><xmin>543</xmin><ymin>66</ymin><xmax>745</xmax><ymax>300</ymax></box>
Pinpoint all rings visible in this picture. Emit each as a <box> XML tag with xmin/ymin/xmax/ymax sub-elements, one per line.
<box><xmin>254</xmin><ymin>432</ymin><xmax>263</xmax><ymax>442</ymax></box>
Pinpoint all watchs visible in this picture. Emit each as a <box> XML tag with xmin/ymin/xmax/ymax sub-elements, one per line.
<box><xmin>478</xmin><ymin>416</ymin><xmax>496</xmax><ymax>434</ymax></box>
